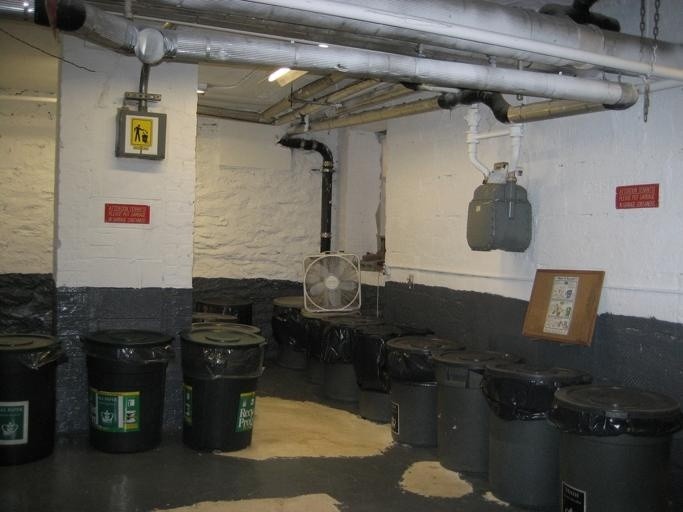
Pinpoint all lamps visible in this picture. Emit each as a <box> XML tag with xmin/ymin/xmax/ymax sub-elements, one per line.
<box><xmin>268</xmin><ymin>66</ymin><xmax>289</xmax><ymax>82</ymax></box>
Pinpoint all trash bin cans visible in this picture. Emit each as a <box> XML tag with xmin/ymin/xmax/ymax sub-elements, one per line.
<box><xmin>320</xmin><ymin>316</ymin><xmax>392</xmax><ymax>403</ymax></box>
<box><xmin>0</xmin><ymin>334</ymin><xmax>61</xmax><ymax>466</ymax></box>
<box><xmin>553</xmin><ymin>383</ymin><xmax>682</xmax><ymax>512</ymax></box>
<box><xmin>350</xmin><ymin>323</ymin><xmax>434</xmax><ymax>424</ymax></box>
<box><xmin>300</xmin><ymin>307</ymin><xmax>352</xmax><ymax>380</ymax></box>
<box><xmin>386</xmin><ymin>337</ymin><xmax>467</xmax><ymax>447</ymax></box>
<box><xmin>79</xmin><ymin>329</ymin><xmax>174</xmax><ymax>452</ymax></box>
<box><xmin>179</xmin><ymin>298</ymin><xmax>266</xmax><ymax>452</ymax></box>
<box><xmin>428</xmin><ymin>347</ymin><xmax>513</xmax><ymax>474</ymax></box>
<box><xmin>273</xmin><ymin>297</ymin><xmax>324</xmax><ymax>372</ymax></box>
<box><xmin>484</xmin><ymin>362</ymin><xmax>592</xmax><ymax>511</ymax></box>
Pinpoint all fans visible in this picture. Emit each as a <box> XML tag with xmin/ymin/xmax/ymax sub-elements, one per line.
<box><xmin>303</xmin><ymin>251</ymin><xmax>362</xmax><ymax>313</ymax></box>
